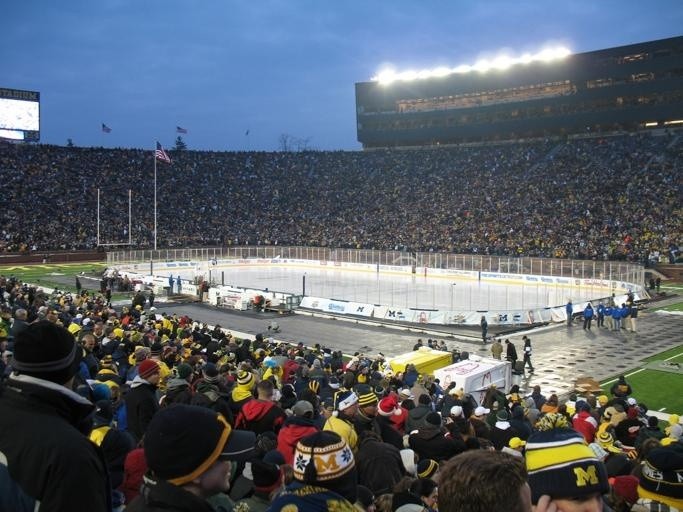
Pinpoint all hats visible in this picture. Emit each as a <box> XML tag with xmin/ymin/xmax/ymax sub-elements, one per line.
<box><xmin>204</xmin><ymin>369</ymin><xmax>220</xmax><ymax>384</ymax></box>
<box><xmin>151</xmin><ymin>342</ymin><xmax>164</xmax><ymax>356</ymax></box>
<box><xmin>291</xmin><ymin>400</ymin><xmax>315</xmax><ymax>418</ymax></box>
<box><xmin>496</xmin><ymin>410</ymin><xmax>507</xmax><ymax>420</ymax></box>
<box><xmin>450</xmin><ymin>405</ymin><xmax>463</xmax><ymax>417</ymax></box>
<box><xmin>417</xmin><ymin>459</ymin><xmax>439</xmax><ymax>480</ymax></box>
<box><xmin>177</xmin><ymin>363</ymin><xmax>193</xmax><ymax>378</ymax></box>
<box><xmin>138</xmin><ymin>360</ymin><xmax>160</xmax><ymax>378</ymax></box>
<box><xmin>293</xmin><ymin>430</ymin><xmax>357</xmax><ymax>504</ymax></box>
<box><xmin>475</xmin><ymin>406</ymin><xmax>491</xmax><ymax>417</ymax></box>
<box><xmin>10</xmin><ymin>318</ymin><xmax>82</xmax><ymax>385</ymax></box>
<box><xmin>509</xmin><ymin>412</ymin><xmax>683</xmax><ymax>509</ymax></box>
<box><xmin>251</xmin><ymin>458</ymin><xmax>282</xmax><ymax>491</ymax></box>
<box><xmin>424</xmin><ymin>412</ymin><xmax>441</xmax><ymax>430</ymax></box>
<box><xmin>237</xmin><ymin>369</ymin><xmax>256</xmax><ymax>390</ymax></box>
<box><xmin>143</xmin><ymin>404</ymin><xmax>258</xmax><ymax>490</ymax></box>
<box><xmin>332</xmin><ymin>387</ymin><xmax>411</xmax><ymax>416</ymax></box>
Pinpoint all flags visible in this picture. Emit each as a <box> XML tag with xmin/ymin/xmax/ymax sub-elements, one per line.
<box><xmin>154</xmin><ymin>137</ymin><xmax>171</xmax><ymax>163</ymax></box>
<box><xmin>175</xmin><ymin>126</ymin><xmax>187</xmax><ymax>133</ymax></box>
<box><xmin>102</xmin><ymin>123</ymin><xmax>111</xmax><ymax>133</ymax></box>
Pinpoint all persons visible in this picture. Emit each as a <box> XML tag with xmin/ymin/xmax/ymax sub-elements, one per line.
<box><xmin>1</xmin><ymin>141</ymin><xmax>682</xmax><ymax>264</ymax></box>
<box><xmin>1</xmin><ymin>275</ymin><xmax>682</xmax><ymax>512</ymax></box>
